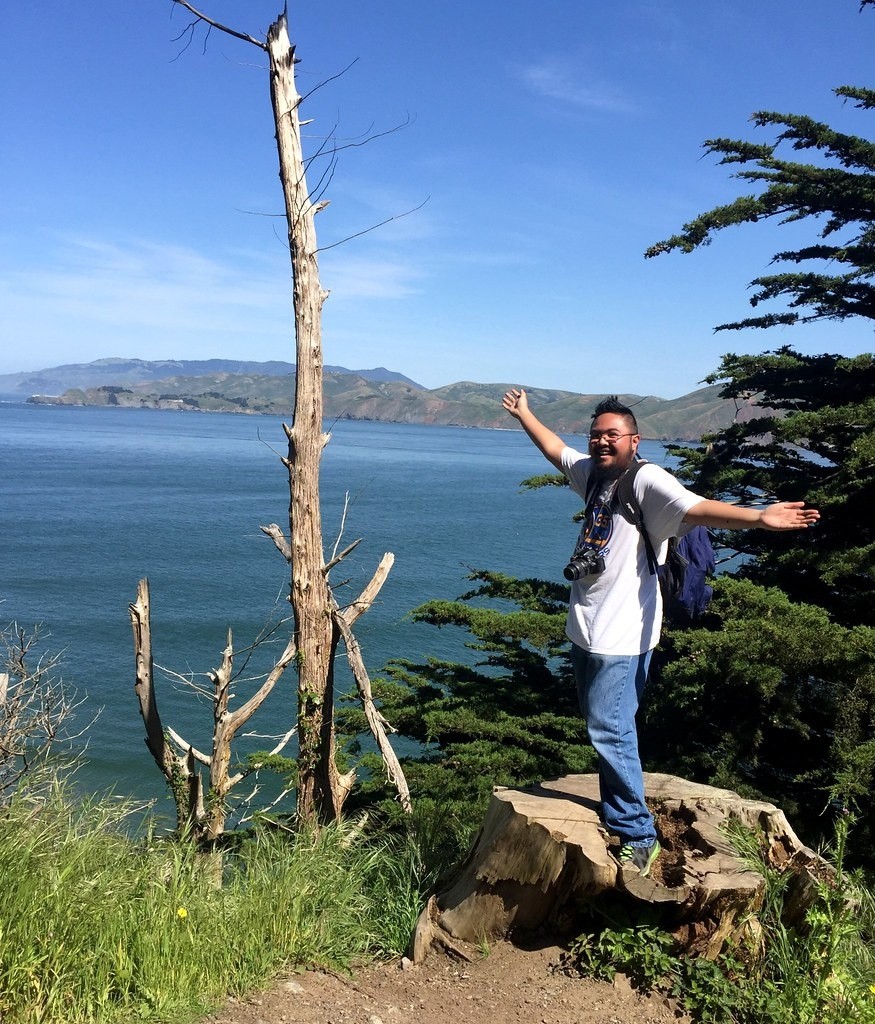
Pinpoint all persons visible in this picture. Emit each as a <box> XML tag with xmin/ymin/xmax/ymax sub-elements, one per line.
<box><xmin>502</xmin><ymin>388</ymin><xmax>821</xmax><ymax>877</ymax></box>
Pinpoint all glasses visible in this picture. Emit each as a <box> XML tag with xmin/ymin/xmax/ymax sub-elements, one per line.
<box><xmin>586</xmin><ymin>433</ymin><xmax>637</xmax><ymax>443</ymax></box>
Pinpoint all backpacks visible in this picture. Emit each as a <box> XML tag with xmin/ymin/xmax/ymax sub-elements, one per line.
<box><xmin>585</xmin><ymin>462</ymin><xmax>714</xmax><ymax>621</ymax></box>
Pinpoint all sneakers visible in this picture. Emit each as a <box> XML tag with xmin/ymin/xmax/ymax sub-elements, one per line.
<box><xmin>616</xmin><ymin>838</ymin><xmax>661</xmax><ymax>877</ymax></box>
